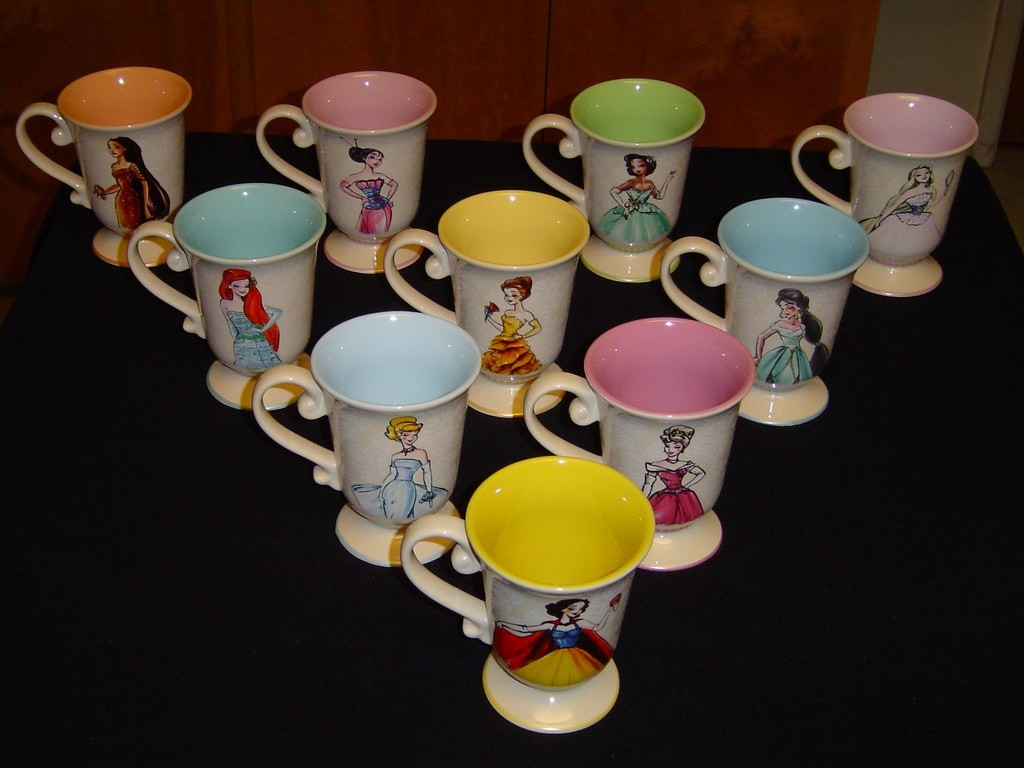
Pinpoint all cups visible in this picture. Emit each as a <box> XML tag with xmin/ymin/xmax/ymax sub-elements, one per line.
<box><xmin>660</xmin><ymin>196</ymin><xmax>870</xmax><ymax>427</ymax></box>
<box><xmin>522</xmin><ymin>318</ymin><xmax>756</xmax><ymax>571</ymax></box>
<box><xmin>255</xmin><ymin>70</ymin><xmax>437</xmax><ymax>276</ymax></box>
<box><xmin>523</xmin><ymin>78</ymin><xmax>706</xmax><ymax>283</ymax></box>
<box><xmin>127</xmin><ymin>183</ymin><xmax>327</xmax><ymax>412</ymax></box>
<box><xmin>793</xmin><ymin>93</ymin><xmax>980</xmax><ymax>297</ymax></box>
<box><xmin>15</xmin><ymin>67</ymin><xmax>193</xmax><ymax>268</ymax></box>
<box><xmin>383</xmin><ymin>189</ymin><xmax>591</xmax><ymax>418</ymax></box>
<box><xmin>251</xmin><ymin>310</ymin><xmax>483</xmax><ymax>567</ymax></box>
<box><xmin>401</xmin><ymin>456</ymin><xmax>656</xmax><ymax>734</ymax></box>
